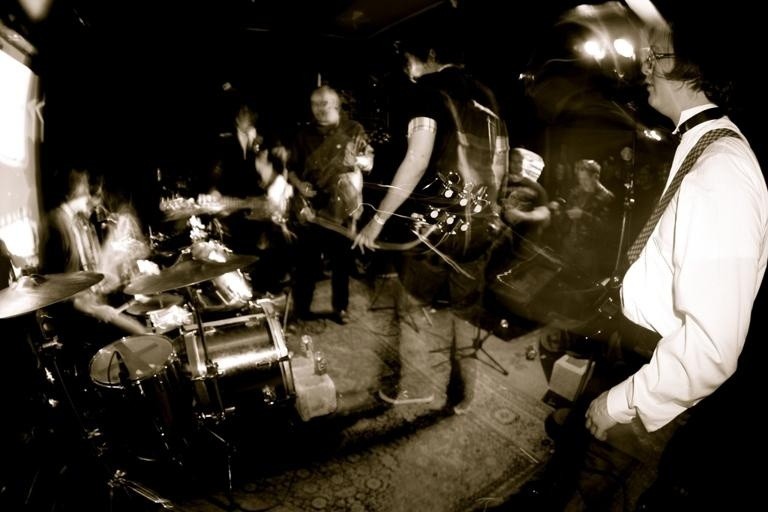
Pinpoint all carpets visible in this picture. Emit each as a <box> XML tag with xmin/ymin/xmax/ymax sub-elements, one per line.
<box><xmin>237</xmin><ymin>292</ymin><xmax>543</xmax><ymax>512</ymax></box>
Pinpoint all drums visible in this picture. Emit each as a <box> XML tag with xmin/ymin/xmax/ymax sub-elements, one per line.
<box><xmin>168</xmin><ymin>239</ymin><xmax>254</xmax><ymax>313</ymax></box>
<box><xmin>178</xmin><ymin>298</ymin><xmax>299</xmax><ymax>427</ymax></box>
<box><xmin>87</xmin><ymin>333</ymin><xmax>194</xmax><ymax>431</ymax></box>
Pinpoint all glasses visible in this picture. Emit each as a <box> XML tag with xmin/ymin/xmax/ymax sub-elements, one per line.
<box><xmin>645</xmin><ymin>51</ymin><xmax>677</xmax><ymax>66</ymax></box>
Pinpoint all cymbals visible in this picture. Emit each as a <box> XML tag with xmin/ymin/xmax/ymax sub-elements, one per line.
<box><xmin>123</xmin><ymin>252</ymin><xmax>261</xmax><ymax>295</ymax></box>
<box><xmin>126</xmin><ymin>292</ymin><xmax>184</xmax><ymax>316</ymax></box>
<box><xmin>0</xmin><ymin>265</ymin><xmax>105</xmax><ymax>318</ymax></box>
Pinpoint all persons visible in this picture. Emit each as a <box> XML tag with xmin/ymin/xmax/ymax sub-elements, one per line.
<box><xmin>551</xmin><ymin>160</ymin><xmax>617</xmax><ymax>273</ymax></box>
<box><xmin>36</xmin><ymin>165</ymin><xmax>155</xmax><ymax>337</ymax></box>
<box><xmin>351</xmin><ymin>32</ymin><xmax>508</xmax><ymax>414</ymax></box>
<box><xmin>503</xmin><ymin>148</ymin><xmax>552</xmax><ymax>260</ymax></box>
<box><xmin>536</xmin><ymin>22</ymin><xmax>768</xmax><ymax>512</ymax></box>
<box><xmin>213</xmin><ymin>106</ymin><xmax>296</xmax><ymax>291</ymax></box>
<box><xmin>287</xmin><ymin>85</ymin><xmax>373</xmax><ymax>324</ymax></box>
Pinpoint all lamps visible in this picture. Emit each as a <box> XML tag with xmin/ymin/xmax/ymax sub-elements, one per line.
<box><xmin>586</xmin><ymin>0</ymin><xmax>636</xmax><ymax>60</ymax></box>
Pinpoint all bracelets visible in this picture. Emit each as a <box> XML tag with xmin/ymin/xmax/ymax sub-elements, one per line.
<box><xmin>373</xmin><ymin>214</ymin><xmax>388</xmax><ymax>226</ymax></box>
